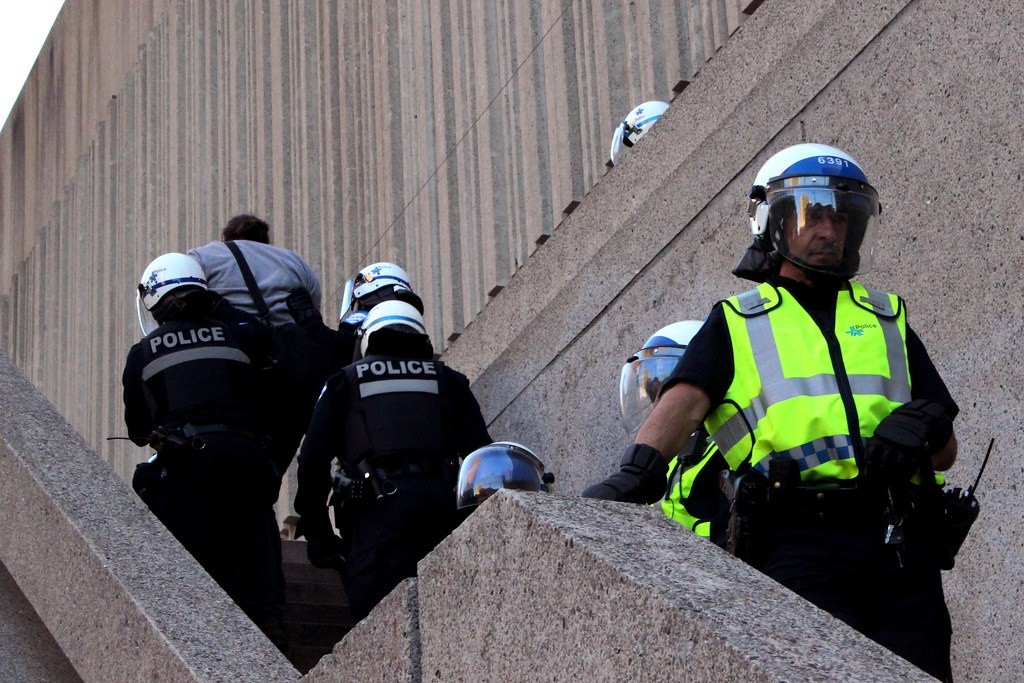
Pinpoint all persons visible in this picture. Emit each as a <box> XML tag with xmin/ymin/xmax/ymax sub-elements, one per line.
<box><xmin>610</xmin><ymin>100</ymin><xmax>670</xmax><ymax>164</ymax></box>
<box><xmin>122</xmin><ymin>252</ymin><xmax>290</xmax><ymax>653</ymax></box>
<box><xmin>187</xmin><ymin>213</ymin><xmax>320</xmax><ymax>473</ymax></box>
<box><xmin>584</xmin><ymin>142</ymin><xmax>959</xmax><ymax>683</ymax></box>
<box><xmin>294</xmin><ymin>300</ymin><xmax>494</xmax><ymax>621</ymax></box>
<box><xmin>619</xmin><ymin>320</ymin><xmax>710</xmax><ymax>538</ymax></box>
<box><xmin>285</xmin><ymin>263</ymin><xmax>423</xmax><ymax>364</ymax></box>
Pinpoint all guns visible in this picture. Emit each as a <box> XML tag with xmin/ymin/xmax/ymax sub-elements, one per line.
<box><xmin>716</xmin><ymin>469</ymin><xmax>762</xmax><ymax>561</ymax></box>
<box><xmin>149</xmin><ymin>424</ymin><xmax>190</xmax><ymax>449</ymax></box>
<box><xmin>337</xmin><ymin>466</ymin><xmax>365</xmax><ymax>538</ymax></box>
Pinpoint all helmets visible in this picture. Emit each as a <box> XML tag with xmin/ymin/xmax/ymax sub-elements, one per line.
<box><xmin>610</xmin><ymin>101</ymin><xmax>670</xmax><ymax>166</ymax></box>
<box><xmin>456</xmin><ymin>441</ymin><xmax>555</xmax><ymax>510</ymax></box>
<box><xmin>618</xmin><ymin>319</ymin><xmax>705</xmax><ymax>441</ymax></box>
<box><xmin>136</xmin><ymin>252</ymin><xmax>208</xmax><ymax>337</ymax></box>
<box><xmin>353</xmin><ymin>300</ymin><xmax>426</xmax><ymax>359</ymax></box>
<box><xmin>746</xmin><ymin>142</ymin><xmax>883</xmax><ymax>276</ymax></box>
<box><xmin>340</xmin><ymin>262</ymin><xmax>413</xmax><ymax>321</ymax></box>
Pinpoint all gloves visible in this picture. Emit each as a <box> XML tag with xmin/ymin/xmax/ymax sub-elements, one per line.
<box><xmin>581</xmin><ymin>443</ymin><xmax>669</xmax><ymax>506</ymax></box>
<box><xmin>864</xmin><ymin>398</ymin><xmax>953</xmax><ymax>487</ymax></box>
<box><xmin>286</xmin><ymin>285</ymin><xmax>316</xmax><ymax>325</ymax></box>
<box><xmin>294</xmin><ymin>507</ymin><xmax>348</xmax><ymax>570</ymax></box>
